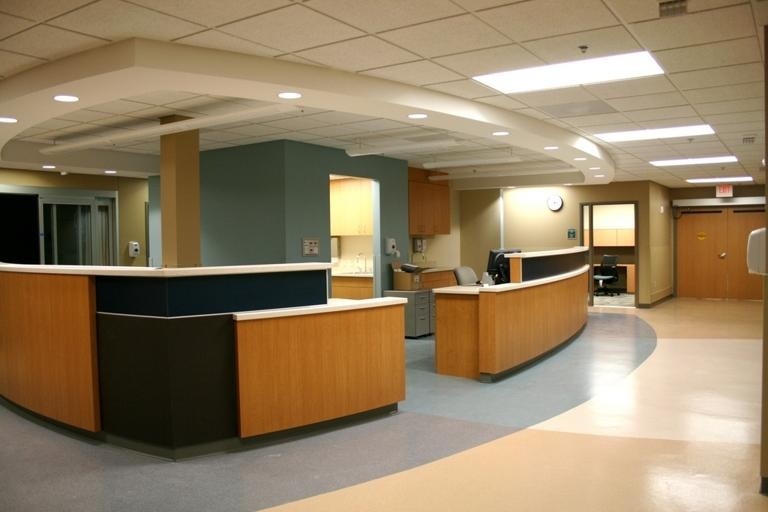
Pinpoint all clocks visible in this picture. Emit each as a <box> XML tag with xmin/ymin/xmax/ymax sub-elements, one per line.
<box><xmin>547</xmin><ymin>195</ymin><xmax>563</xmax><ymax>211</ymax></box>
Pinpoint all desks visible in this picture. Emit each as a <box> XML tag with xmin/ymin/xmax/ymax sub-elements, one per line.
<box><xmin>430</xmin><ymin>246</ymin><xmax>592</xmax><ymax>383</ymax></box>
<box><xmin>0</xmin><ymin>263</ymin><xmax>407</xmax><ymax>460</ymax></box>
<box><xmin>592</xmin><ymin>264</ymin><xmax>635</xmax><ymax>294</ymax></box>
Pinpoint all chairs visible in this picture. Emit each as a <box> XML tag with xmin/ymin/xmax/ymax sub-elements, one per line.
<box><xmin>594</xmin><ymin>255</ymin><xmax>620</xmax><ymax>297</ymax></box>
<box><xmin>452</xmin><ymin>265</ymin><xmax>479</xmax><ymax>285</ymax></box>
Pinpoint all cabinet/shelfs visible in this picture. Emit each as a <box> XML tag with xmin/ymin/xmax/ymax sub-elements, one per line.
<box><xmin>393</xmin><ymin>267</ymin><xmax>459</xmax><ymax>289</ymax></box>
<box><xmin>583</xmin><ymin>228</ymin><xmax>636</xmax><ymax>248</ymax></box>
<box><xmin>332</xmin><ymin>277</ymin><xmax>374</xmax><ymax>301</ymax></box>
<box><xmin>408</xmin><ymin>167</ymin><xmax>452</xmax><ymax>237</ymax></box>
<box><xmin>383</xmin><ymin>290</ymin><xmax>436</xmax><ymax>338</ymax></box>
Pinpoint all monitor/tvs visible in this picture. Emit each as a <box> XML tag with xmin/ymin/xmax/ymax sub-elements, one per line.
<box><xmin>487</xmin><ymin>248</ymin><xmax>522</xmax><ymax>284</ymax></box>
